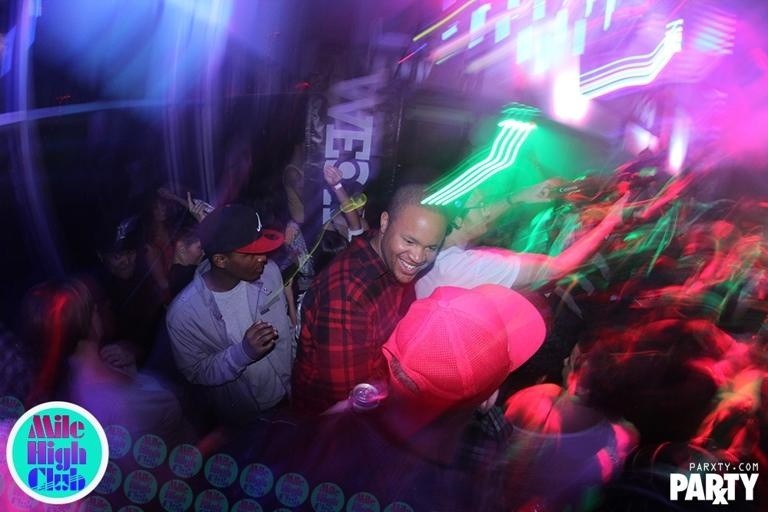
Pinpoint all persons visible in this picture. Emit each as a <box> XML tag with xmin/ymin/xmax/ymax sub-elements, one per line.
<box><xmin>276</xmin><ymin>137</ymin><xmax>306</xmax><ymax>221</ymax></box>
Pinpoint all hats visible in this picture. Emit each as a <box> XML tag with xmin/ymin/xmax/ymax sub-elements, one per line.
<box><xmin>197</xmin><ymin>204</ymin><xmax>287</xmax><ymax>255</ymax></box>
<box><xmin>382</xmin><ymin>281</ymin><xmax>548</xmax><ymax>420</ymax></box>
<box><xmin>102</xmin><ymin>210</ymin><xmax>143</xmax><ymax>251</ymax></box>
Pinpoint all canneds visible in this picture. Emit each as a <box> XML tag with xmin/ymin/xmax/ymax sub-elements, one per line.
<box><xmin>350</xmin><ymin>384</ymin><xmax>381</xmax><ymax>411</ymax></box>
